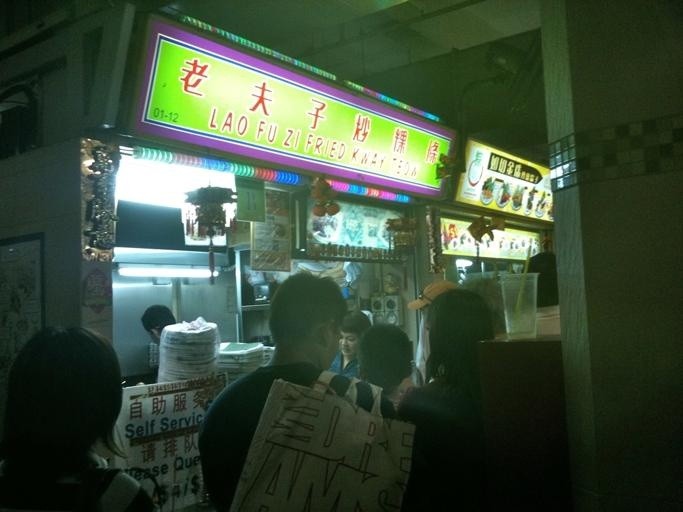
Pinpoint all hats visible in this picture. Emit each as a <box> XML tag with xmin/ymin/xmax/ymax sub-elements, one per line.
<box><xmin>407</xmin><ymin>282</ymin><xmax>458</xmax><ymax>309</ymax></box>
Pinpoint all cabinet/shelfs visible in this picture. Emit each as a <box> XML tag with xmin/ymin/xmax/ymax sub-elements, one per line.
<box><xmin>477</xmin><ymin>338</ymin><xmax>572</xmax><ymax>512</ymax></box>
<box><xmin>237</xmin><ymin>244</ymin><xmax>374</xmax><ymax>368</ymax></box>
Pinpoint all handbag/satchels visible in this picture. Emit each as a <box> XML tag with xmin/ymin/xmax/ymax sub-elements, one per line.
<box><xmin>231</xmin><ymin>371</ymin><xmax>416</xmax><ymax>511</ymax></box>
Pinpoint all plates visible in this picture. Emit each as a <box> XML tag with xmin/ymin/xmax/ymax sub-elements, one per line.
<box><xmin>219</xmin><ymin>342</ymin><xmax>264</xmax><ymax>369</ymax></box>
<box><xmin>158</xmin><ymin>322</ymin><xmax>216</xmax><ymax>382</ymax></box>
<box><xmin>482</xmin><ymin>181</ymin><xmax>556</xmax><ymax>219</ymax></box>
<box><xmin>264</xmin><ymin>347</ymin><xmax>275</xmax><ymax>362</ymax></box>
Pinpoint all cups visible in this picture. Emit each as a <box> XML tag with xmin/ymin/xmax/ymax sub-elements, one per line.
<box><xmin>497</xmin><ymin>272</ymin><xmax>540</xmax><ymax>339</ymax></box>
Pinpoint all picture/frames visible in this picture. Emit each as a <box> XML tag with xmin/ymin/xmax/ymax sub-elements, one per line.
<box><xmin>0</xmin><ymin>232</ymin><xmax>47</xmax><ymax>409</ymax></box>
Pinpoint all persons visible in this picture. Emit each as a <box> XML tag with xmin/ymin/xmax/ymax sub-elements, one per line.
<box><xmin>3</xmin><ymin>322</ymin><xmax>158</xmax><ymax>511</ymax></box>
<box><xmin>198</xmin><ymin>270</ymin><xmax>428</xmax><ymax>511</ymax></box>
<box><xmin>139</xmin><ymin>302</ymin><xmax>177</xmax><ymax>345</ymax></box>
<box><xmin>324</xmin><ymin>252</ymin><xmax>562</xmax><ymax>510</ymax></box>
